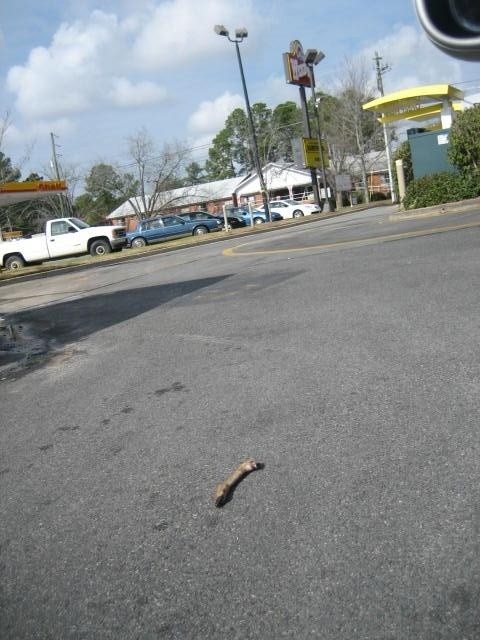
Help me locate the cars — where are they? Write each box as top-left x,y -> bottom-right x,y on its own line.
254,199 -> 322,219
217,205 -> 284,225
177,211 -> 247,232
126,214 -> 223,249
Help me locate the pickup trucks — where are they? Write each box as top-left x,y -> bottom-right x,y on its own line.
0,216 -> 129,272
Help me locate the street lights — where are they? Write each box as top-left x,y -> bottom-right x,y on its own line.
303,47 -> 336,213
212,24 -> 271,223
371,51 -> 400,204
